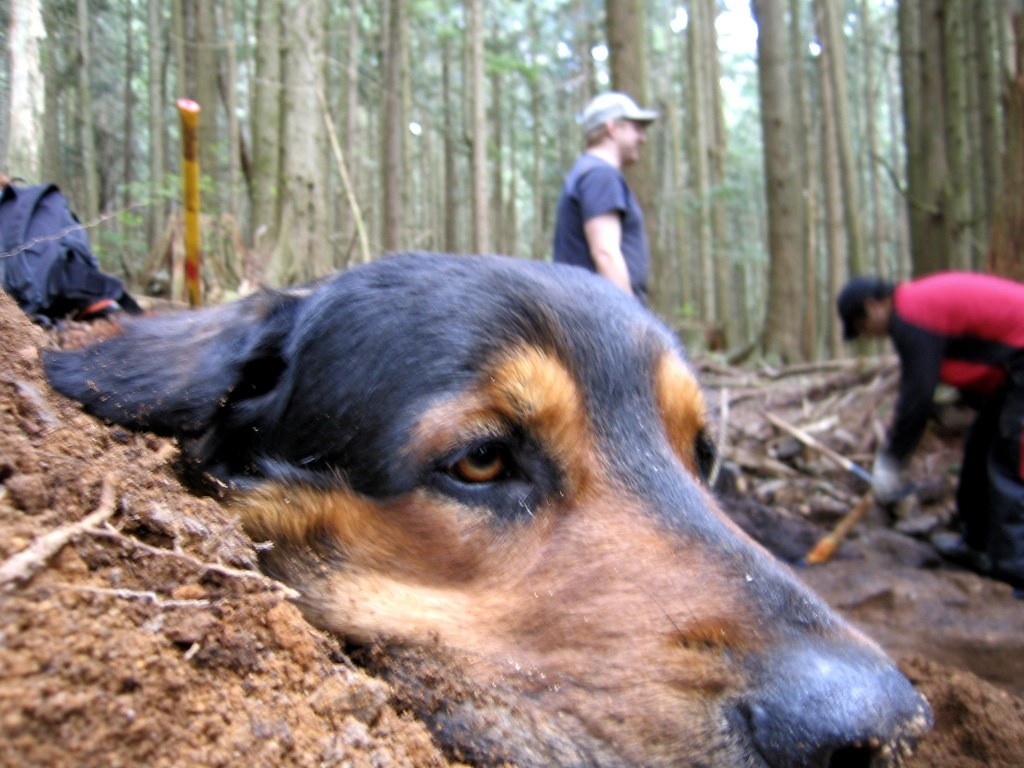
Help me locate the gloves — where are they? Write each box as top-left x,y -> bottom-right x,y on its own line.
872,454 -> 908,507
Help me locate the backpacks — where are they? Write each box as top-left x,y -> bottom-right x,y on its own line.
1,180 -> 100,320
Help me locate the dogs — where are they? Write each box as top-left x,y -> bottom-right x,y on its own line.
32,253 -> 937,768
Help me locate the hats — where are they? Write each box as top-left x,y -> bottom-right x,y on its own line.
836,278 -> 893,339
582,93 -> 655,132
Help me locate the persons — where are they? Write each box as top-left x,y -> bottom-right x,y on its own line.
837,271 -> 1024,600
552,91 -> 658,308
